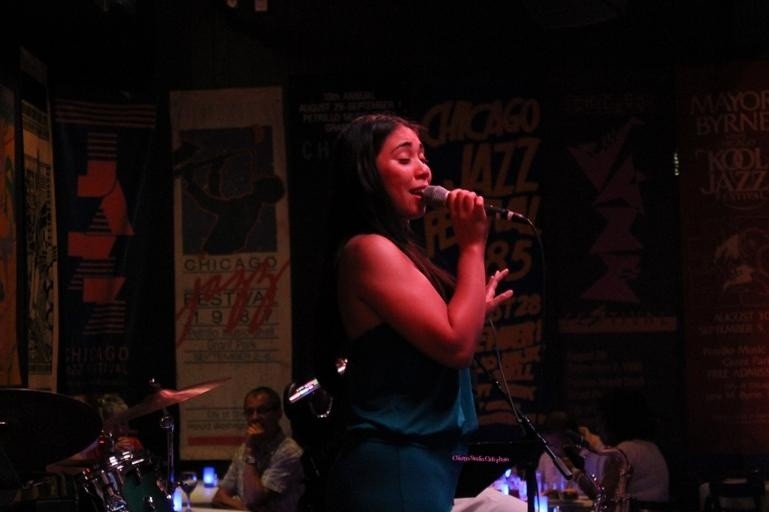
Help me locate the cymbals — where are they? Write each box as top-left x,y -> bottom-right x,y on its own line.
0,388 -> 104,472
123,378 -> 231,421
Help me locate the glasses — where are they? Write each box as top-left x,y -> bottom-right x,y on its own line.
243,405 -> 274,417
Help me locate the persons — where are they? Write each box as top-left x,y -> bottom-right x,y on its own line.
211,385 -> 306,512
97,391 -> 145,455
537,415 -> 602,496
590,391 -> 671,512
296,113 -> 515,512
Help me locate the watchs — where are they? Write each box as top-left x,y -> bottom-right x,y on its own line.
244,455 -> 258,464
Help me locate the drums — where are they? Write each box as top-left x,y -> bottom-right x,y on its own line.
73,451 -> 155,512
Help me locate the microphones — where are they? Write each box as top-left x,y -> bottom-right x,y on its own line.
422,185 -> 525,223
